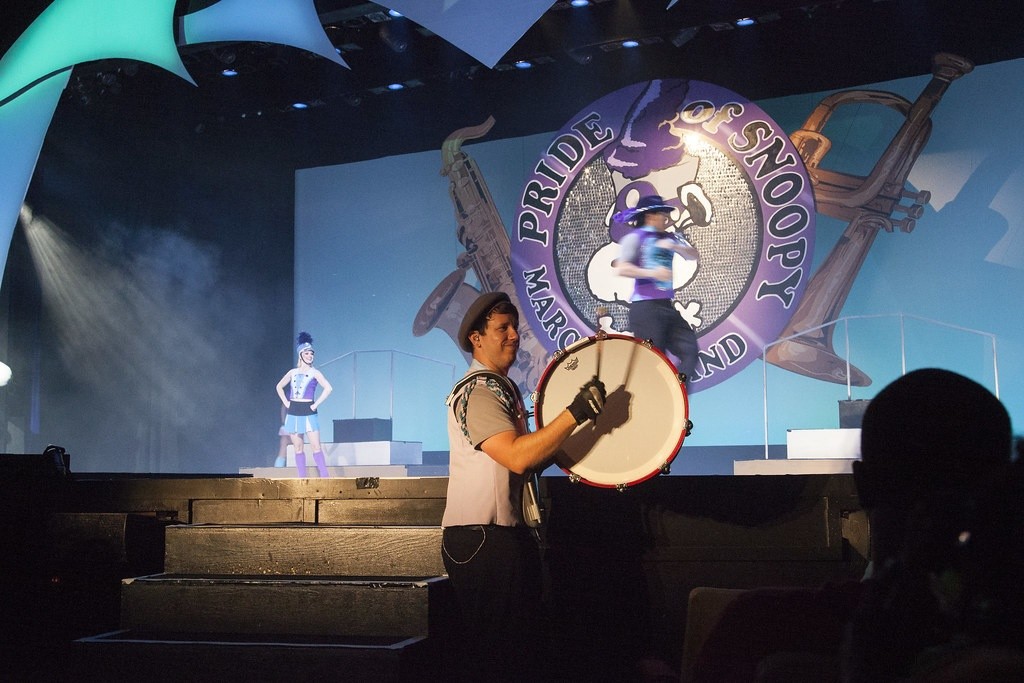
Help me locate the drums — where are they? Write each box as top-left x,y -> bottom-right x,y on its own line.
530,333 -> 694,491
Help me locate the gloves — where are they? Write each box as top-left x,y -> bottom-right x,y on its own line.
565,375 -> 607,427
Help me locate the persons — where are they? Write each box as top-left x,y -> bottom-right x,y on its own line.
619,196 -> 699,383
441,292 -> 605,683
688,368 -> 1024,683
276,332 -> 333,477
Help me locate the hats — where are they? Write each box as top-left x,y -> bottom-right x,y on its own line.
296,330 -> 316,356
621,195 -> 677,222
456,290 -> 512,353
